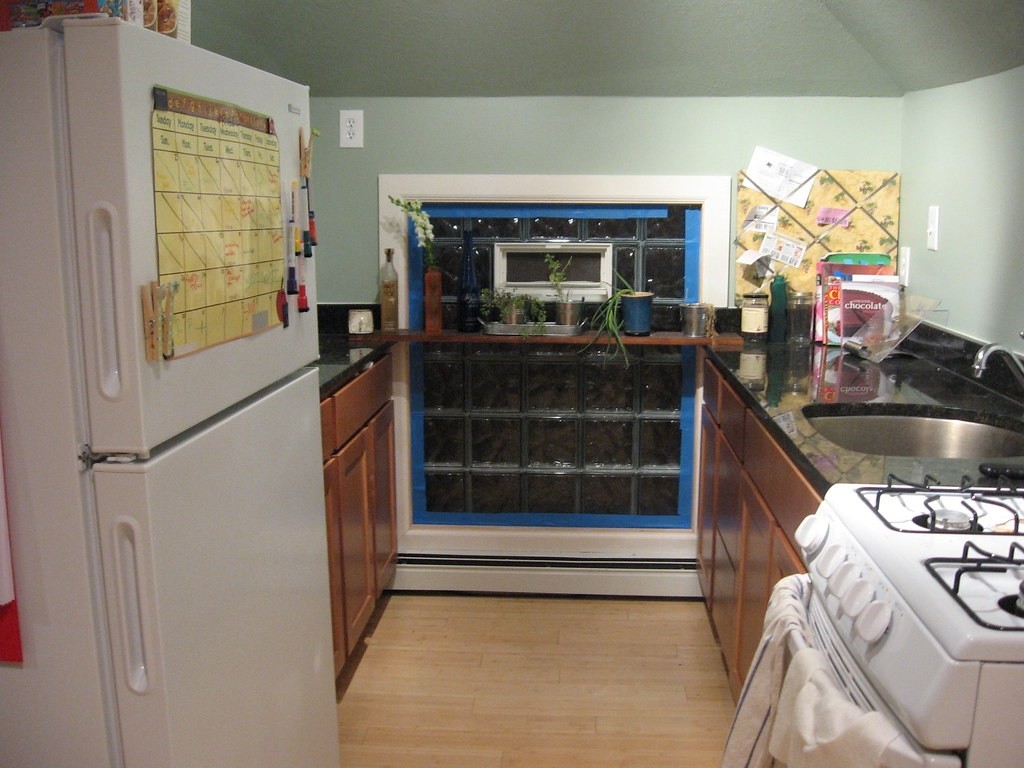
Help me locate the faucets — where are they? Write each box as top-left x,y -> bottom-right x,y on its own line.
971,342 -> 1024,395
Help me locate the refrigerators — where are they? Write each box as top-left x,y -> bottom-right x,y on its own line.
0,13 -> 342,768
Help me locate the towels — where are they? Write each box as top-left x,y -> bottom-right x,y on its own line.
764,648 -> 901,768
720,572 -> 813,768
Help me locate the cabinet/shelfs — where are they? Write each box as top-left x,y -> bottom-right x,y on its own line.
698,358 -> 822,707
411,341 -> 692,529
319,354 -> 398,678
407,201 -> 701,334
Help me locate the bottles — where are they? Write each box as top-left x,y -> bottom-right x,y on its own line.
441,296 -> 458,329
458,219 -> 480,333
770,276 -> 787,342
790,292 -> 814,345
741,293 -> 768,344
736,345 -> 766,392
380,249 -> 398,332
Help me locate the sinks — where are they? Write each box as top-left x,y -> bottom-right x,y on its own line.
795,403 -> 1024,457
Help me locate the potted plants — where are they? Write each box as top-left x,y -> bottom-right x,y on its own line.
578,268 -> 654,368
544,253 -> 582,324
481,288 -> 547,339
388,195 -> 443,333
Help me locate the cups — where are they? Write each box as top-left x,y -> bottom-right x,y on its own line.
348,309 -> 374,333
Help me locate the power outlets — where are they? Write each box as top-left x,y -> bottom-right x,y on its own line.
338,109 -> 363,148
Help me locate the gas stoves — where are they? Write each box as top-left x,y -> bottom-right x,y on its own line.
834,463 -> 1024,748
